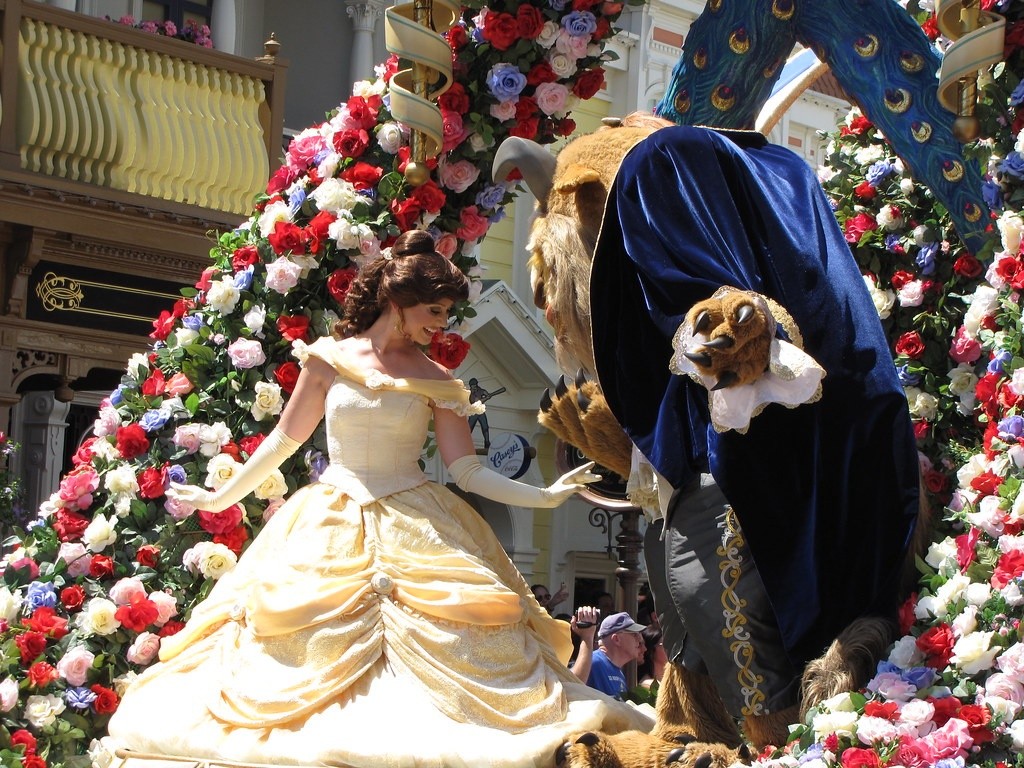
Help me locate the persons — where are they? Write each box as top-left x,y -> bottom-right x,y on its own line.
529,581 -> 671,707
91,230 -> 667,767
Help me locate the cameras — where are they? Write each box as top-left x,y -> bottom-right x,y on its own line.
574,609 -> 601,628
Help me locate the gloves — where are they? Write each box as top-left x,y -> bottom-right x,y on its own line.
445,454 -> 603,509
164,424 -> 303,514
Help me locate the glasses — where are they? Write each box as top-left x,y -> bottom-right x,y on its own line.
535,593 -> 552,603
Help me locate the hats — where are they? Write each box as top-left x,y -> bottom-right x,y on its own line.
595,611 -> 647,642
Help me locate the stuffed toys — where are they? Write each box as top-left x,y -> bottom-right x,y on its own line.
493,110 -> 936,768
